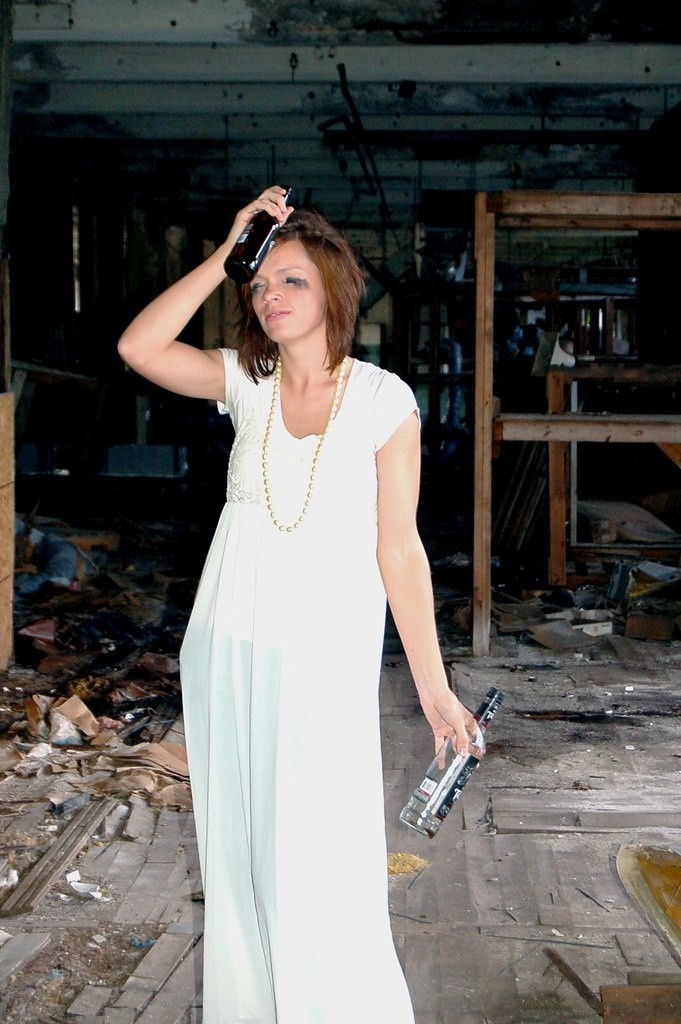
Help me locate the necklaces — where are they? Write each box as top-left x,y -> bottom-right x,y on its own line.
261,355 -> 347,532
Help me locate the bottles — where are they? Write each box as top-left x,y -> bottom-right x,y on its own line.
399,687 -> 506,838
224,185 -> 293,284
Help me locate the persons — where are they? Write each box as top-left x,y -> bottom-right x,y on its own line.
116,186 -> 486,1024
